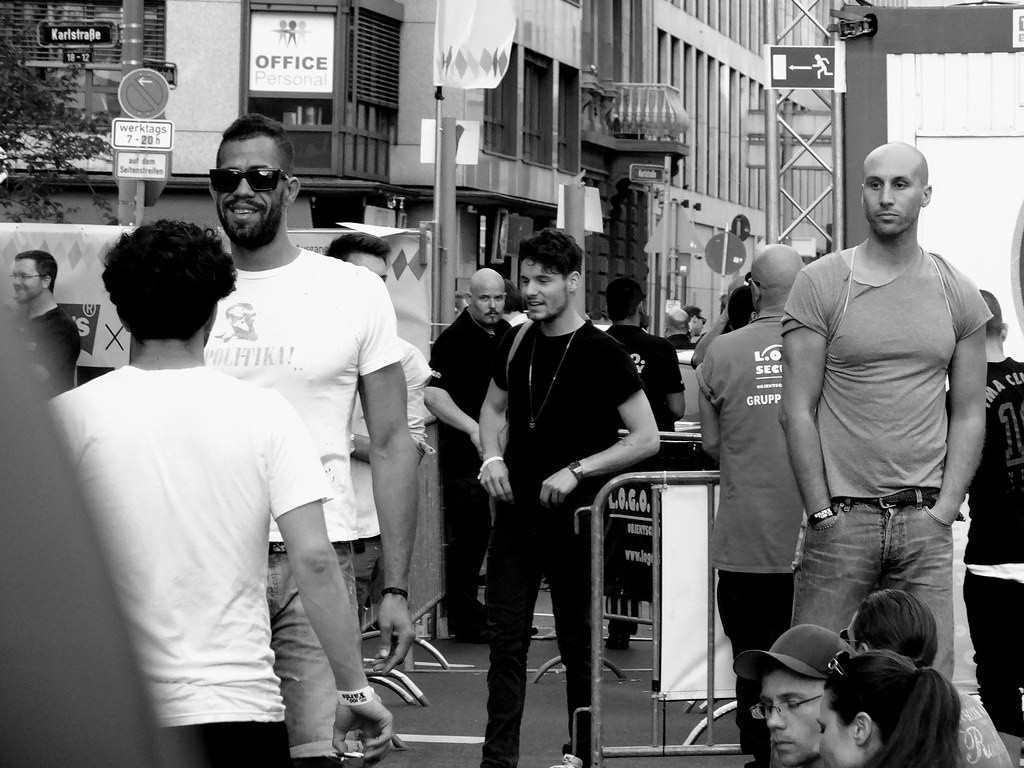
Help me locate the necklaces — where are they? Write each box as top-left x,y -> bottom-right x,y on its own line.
528,325 -> 576,429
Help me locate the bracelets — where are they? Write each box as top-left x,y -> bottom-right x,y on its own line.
808,506 -> 837,526
382,586 -> 408,598
338,684 -> 376,708
478,456 -> 505,480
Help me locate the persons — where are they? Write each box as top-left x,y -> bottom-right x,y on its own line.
479,227 -> 660,768
601,277 -> 687,653
50,220 -> 394,768
665,242 -> 820,768
199,115 -> 415,768
946,288 -> 1024,735
424,268 -> 539,643
322,230 -> 434,632
839,589 -> 1014,768
816,648 -> 960,768
778,141 -> 995,684
733,623 -> 859,768
7,249 -> 82,405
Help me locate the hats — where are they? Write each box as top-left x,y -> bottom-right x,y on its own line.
733,624 -> 859,678
605,279 -> 646,310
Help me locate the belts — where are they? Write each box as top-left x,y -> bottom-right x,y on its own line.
268,541 -> 350,555
830,489 -> 964,522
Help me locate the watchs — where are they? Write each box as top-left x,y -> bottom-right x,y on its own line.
569,461 -> 584,482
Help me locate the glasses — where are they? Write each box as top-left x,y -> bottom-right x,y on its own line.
691,314 -> 706,325
7,273 -> 46,280
209,167 -> 289,193
744,272 -> 760,287
749,695 -> 821,719
840,628 -> 872,650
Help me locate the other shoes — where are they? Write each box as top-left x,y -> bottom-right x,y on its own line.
550,753 -> 582,768
601,632 -> 630,648
478,573 -> 489,588
457,618 -> 538,644
